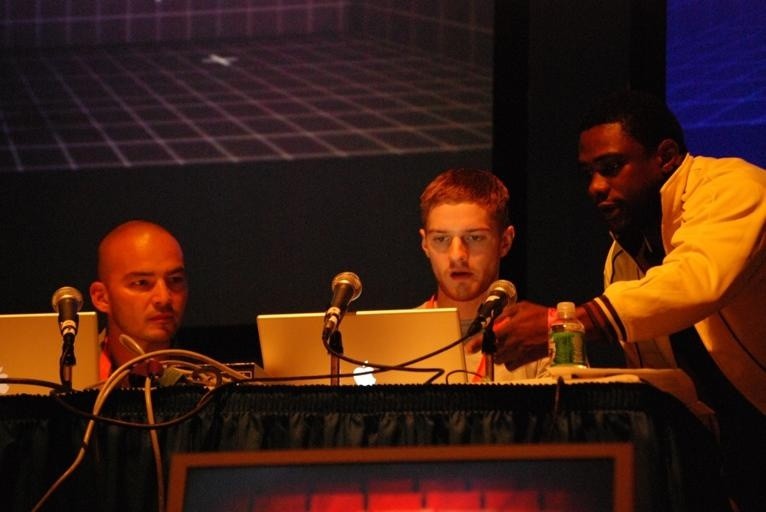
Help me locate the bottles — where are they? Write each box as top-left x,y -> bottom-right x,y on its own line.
549,301 -> 584,365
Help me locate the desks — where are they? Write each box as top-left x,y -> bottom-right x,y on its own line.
0,381 -> 733,511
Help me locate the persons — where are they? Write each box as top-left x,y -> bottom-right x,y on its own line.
467,98 -> 766,512
88,220 -> 188,394
409,164 -> 552,384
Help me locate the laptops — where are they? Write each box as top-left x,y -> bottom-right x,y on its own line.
0,311 -> 100,396
255,308 -> 468,385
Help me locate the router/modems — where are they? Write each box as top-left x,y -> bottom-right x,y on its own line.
167,363 -> 262,386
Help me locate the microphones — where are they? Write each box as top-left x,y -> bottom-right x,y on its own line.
322,271 -> 363,333
52,285 -> 84,364
469,280 -> 517,336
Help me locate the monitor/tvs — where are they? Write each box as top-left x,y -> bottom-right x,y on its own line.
164,443 -> 633,512
